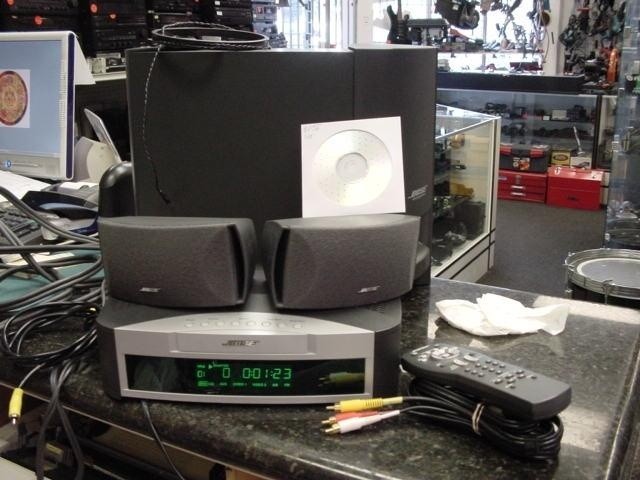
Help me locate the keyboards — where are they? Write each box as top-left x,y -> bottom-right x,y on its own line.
0,205 -> 60,250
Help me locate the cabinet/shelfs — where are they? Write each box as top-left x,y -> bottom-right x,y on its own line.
430,103 -> 502,283
435,86 -> 637,206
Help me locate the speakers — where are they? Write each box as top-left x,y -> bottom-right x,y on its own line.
97,215 -> 259,309
261,213 -> 422,311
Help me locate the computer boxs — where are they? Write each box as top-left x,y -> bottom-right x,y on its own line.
124,46 -> 437,286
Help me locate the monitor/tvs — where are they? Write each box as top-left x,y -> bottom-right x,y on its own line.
1,31 -> 75,181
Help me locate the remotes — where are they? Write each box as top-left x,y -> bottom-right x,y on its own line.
402,342 -> 572,420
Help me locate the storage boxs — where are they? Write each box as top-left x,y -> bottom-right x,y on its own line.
497,170 -> 548,204
546,166 -> 604,210
499,143 -> 550,173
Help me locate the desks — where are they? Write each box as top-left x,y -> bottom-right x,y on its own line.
0,274 -> 640,480
